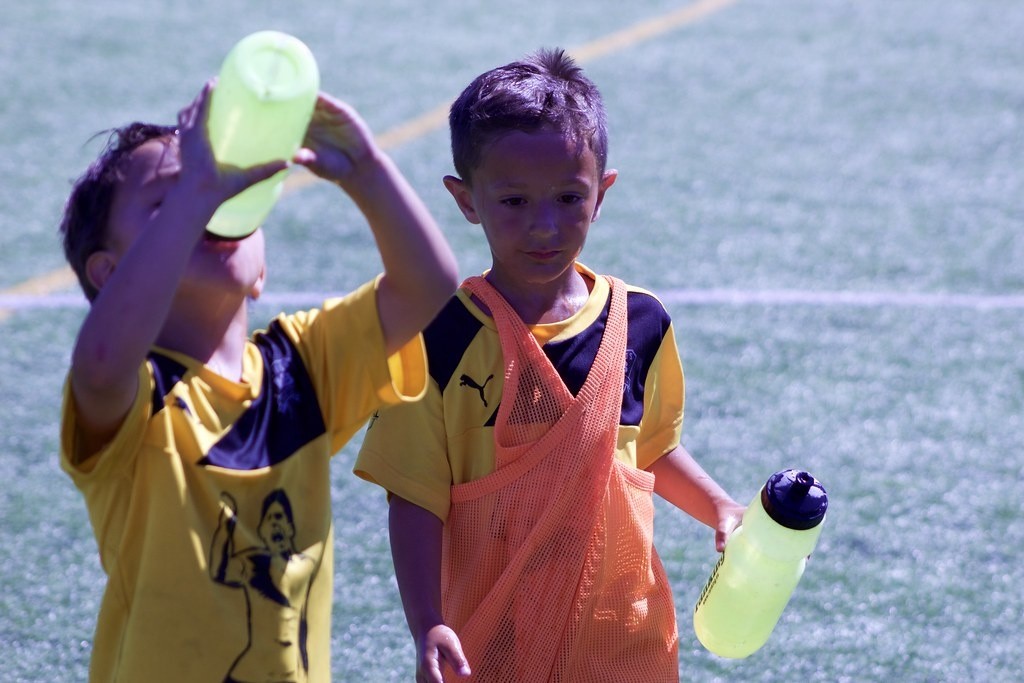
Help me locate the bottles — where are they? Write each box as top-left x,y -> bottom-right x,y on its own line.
206,30 -> 321,242
693,469 -> 830,659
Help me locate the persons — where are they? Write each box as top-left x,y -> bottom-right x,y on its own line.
354,47 -> 750,683
57,81 -> 459,679
